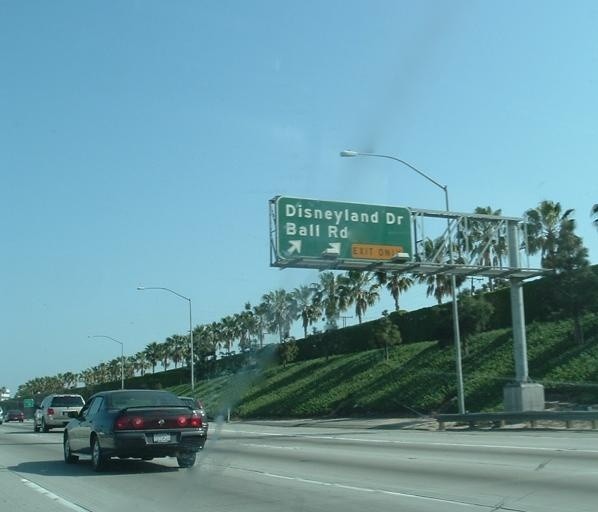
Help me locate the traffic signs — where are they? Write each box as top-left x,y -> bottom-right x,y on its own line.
276,195 -> 413,269
24,399 -> 34,408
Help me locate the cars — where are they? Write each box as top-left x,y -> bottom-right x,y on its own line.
0,406 -> 5,424
4,409 -> 25,423
176,396 -> 209,452
63,387 -> 204,470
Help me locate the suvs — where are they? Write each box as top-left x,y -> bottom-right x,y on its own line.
33,393 -> 86,432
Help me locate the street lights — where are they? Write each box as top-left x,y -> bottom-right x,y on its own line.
340,148 -> 468,420
136,286 -> 195,398
87,335 -> 125,389
